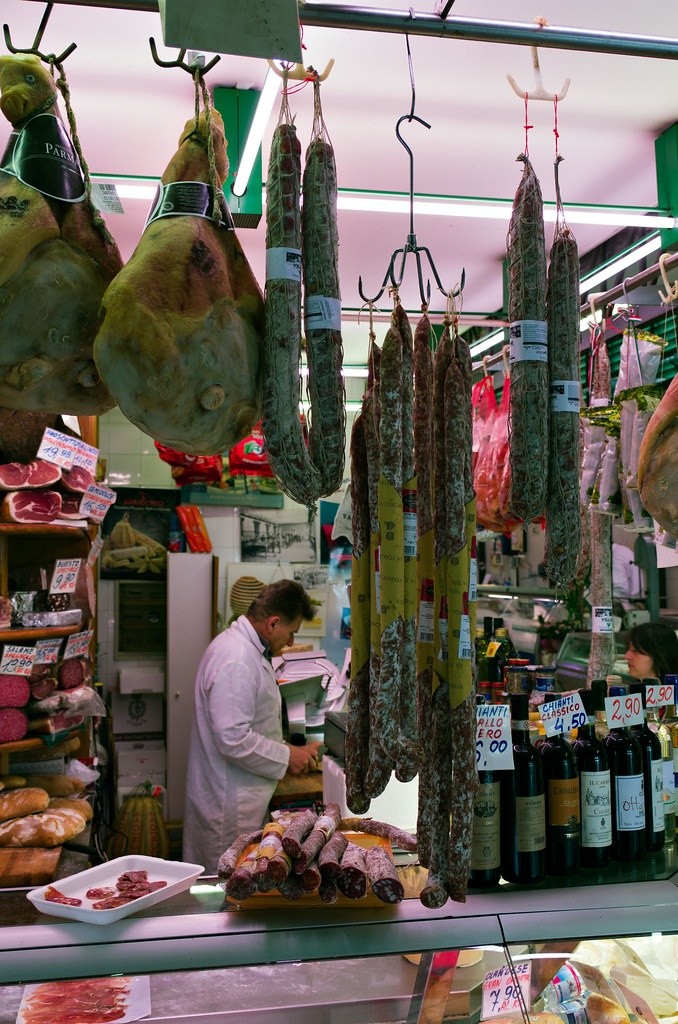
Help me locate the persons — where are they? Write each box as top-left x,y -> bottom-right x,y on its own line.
612,541 -> 643,598
183,579 -> 325,876
624,623 -> 678,678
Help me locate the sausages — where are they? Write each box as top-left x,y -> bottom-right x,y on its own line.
262,123 -> 477,909
216,803 -> 423,904
504,152 -> 614,690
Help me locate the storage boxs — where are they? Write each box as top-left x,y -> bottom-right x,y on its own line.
118,667 -> 165,693
114,741 -> 167,788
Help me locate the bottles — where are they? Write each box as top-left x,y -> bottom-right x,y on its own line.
539,960 -> 603,1024
471,628 -> 678,887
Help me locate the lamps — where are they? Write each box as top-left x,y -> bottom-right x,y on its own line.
232,59 -> 293,197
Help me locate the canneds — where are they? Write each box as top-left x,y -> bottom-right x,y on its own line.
477,658 -> 556,708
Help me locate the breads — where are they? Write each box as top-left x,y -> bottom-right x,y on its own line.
476,993 -> 632,1024
0,772 -> 94,848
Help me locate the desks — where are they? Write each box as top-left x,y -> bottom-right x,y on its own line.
272,772 -> 323,805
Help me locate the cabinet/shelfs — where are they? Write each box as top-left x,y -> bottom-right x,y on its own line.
0,498 -> 97,775
